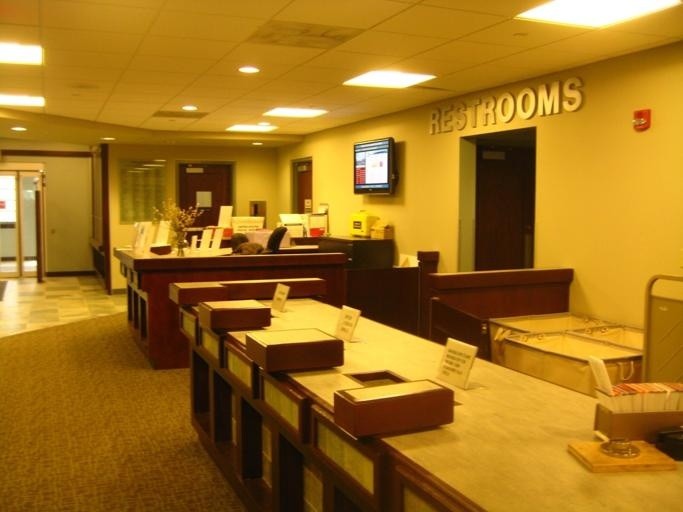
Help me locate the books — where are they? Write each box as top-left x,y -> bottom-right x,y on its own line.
566,438 -> 680,474
593,381 -> 683,415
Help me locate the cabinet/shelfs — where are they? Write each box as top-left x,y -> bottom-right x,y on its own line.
292,234 -> 393,268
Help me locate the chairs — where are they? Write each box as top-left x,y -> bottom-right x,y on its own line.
266,226 -> 288,253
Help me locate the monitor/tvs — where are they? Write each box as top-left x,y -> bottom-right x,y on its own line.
353,134 -> 399,196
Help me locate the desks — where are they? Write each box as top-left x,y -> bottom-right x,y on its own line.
177,296 -> 682,512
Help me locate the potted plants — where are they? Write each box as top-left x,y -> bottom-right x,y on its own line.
151,198 -> 204,257
